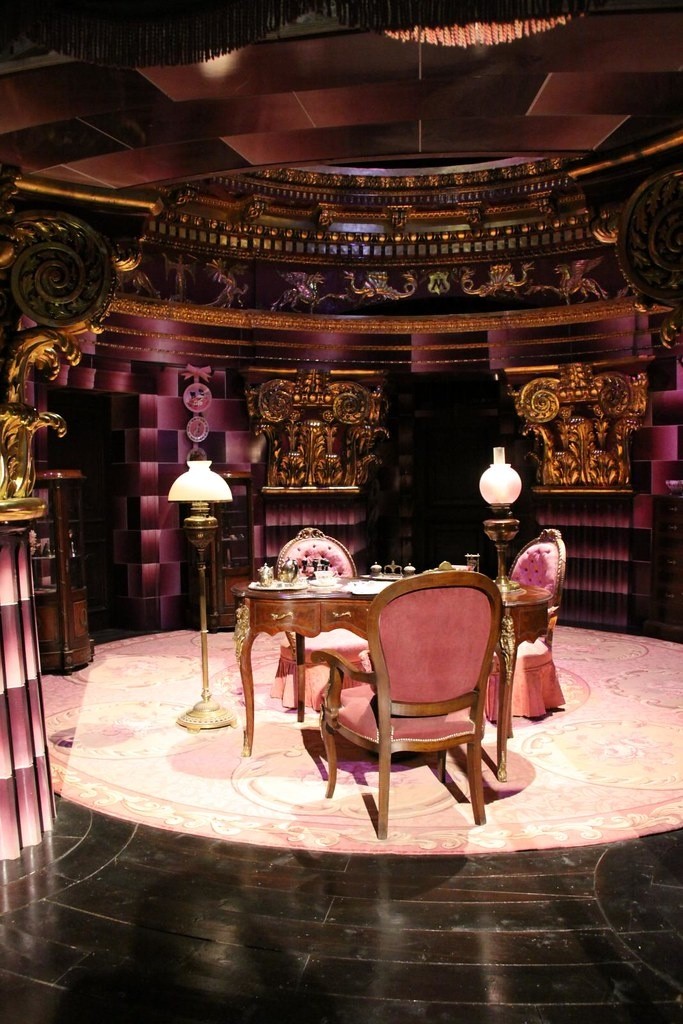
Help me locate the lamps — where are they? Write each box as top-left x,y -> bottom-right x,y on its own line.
167,458 -> 240,731
479,445 -> 525,599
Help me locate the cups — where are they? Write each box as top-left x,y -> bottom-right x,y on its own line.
403,563 -> 416,577
315,571 -> 333,586
370,562 -> 382,576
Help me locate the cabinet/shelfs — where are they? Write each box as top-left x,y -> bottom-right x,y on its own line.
30,467 -> 93,673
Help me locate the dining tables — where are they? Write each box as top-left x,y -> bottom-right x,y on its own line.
228,573 -> 556,785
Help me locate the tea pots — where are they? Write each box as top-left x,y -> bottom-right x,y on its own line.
258,563 -> 274,586
280,557 -> 299,585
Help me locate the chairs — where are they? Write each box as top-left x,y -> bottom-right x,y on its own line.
483,527 -> 568,722
269,526 -> 371,712
308,569 -> 504,842
